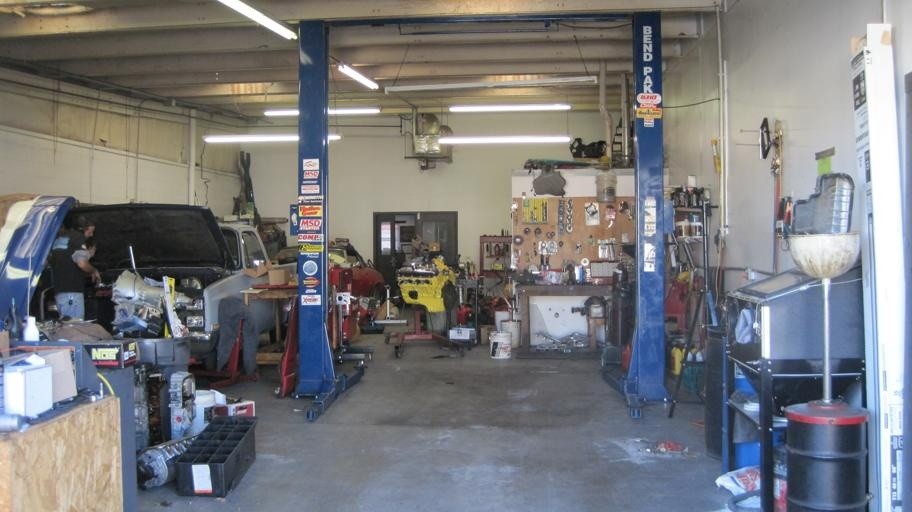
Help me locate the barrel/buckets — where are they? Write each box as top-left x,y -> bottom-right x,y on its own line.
704,325 -> 733,461
722,359 -> 785,472
596,173 -> 617,202
489,331 -> 512,359
502,320 -> 521,349
192,390 -> 216,436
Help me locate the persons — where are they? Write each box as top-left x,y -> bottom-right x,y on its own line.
48,214 -> 102,320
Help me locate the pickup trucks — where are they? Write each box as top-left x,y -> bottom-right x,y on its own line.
6,202 -> 287,381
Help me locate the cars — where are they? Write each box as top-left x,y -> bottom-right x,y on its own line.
266,236 -> 387,327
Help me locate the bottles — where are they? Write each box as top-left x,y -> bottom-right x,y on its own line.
775,195 -> 793,234
668,343 -> 705,376
671,186 -> 705,237
22,316 -> 40,341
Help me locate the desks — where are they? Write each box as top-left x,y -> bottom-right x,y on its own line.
511,280 -> 621,361
